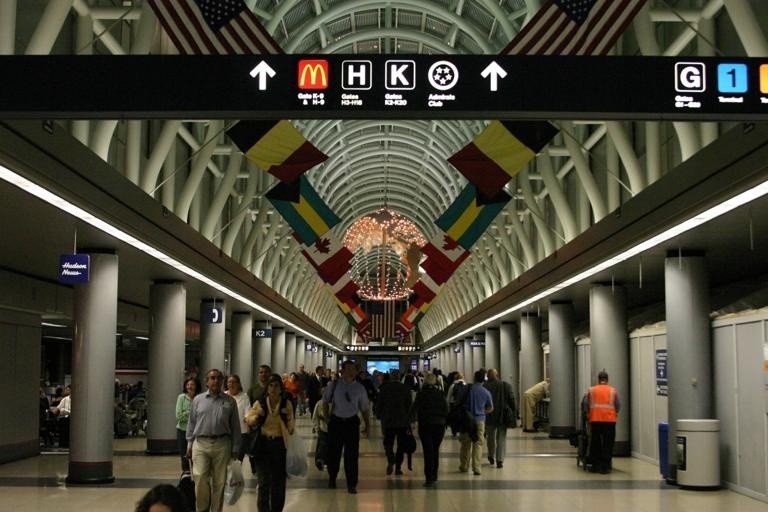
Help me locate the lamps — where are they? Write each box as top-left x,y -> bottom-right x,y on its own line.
342,128 -> 429,302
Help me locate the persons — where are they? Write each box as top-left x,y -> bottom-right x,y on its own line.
176,364 -> 274,480
288,360 -> 518,492
135,481 -> 192,511
113,380 -> 147,436
244,374 -> 295,511
39,379 -> 71,447
580,370 -> 621,473
523,378 -> 551,431
187,369 -> 242,510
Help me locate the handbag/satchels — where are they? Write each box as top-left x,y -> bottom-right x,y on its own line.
503,403 -> 516,428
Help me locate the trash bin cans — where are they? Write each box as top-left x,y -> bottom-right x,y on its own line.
675,418 -> 721,489
658,421 -> 668,479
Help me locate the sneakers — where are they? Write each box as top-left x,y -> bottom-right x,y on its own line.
329,457 -> 503,493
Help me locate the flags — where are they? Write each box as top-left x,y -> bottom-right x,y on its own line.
396,115 -> 558,339
226,119 -> 371,342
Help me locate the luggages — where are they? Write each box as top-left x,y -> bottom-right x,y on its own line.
179,456 -> 211,512
